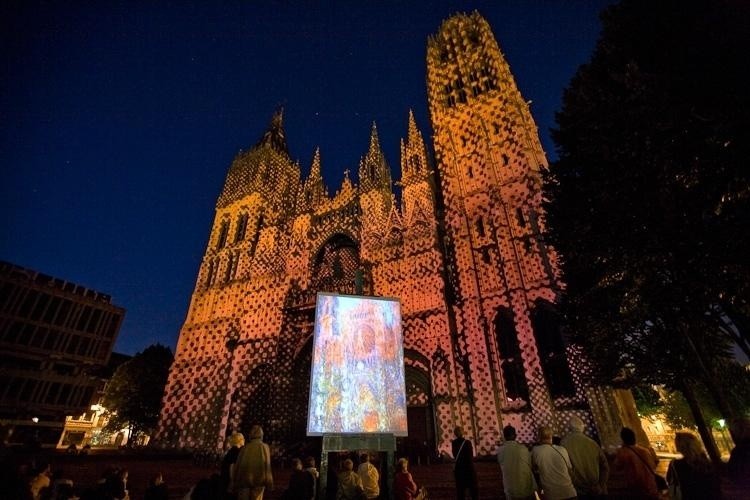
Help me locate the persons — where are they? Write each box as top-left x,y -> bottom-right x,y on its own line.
666,433 -> 714,500
285,454 -> 418,499
531,428 -> 577,500
498,426 -> 534,499
452,427 -> 479,500
726,418 -> 750,485
560,419 -> 610,500
17,417 -> 274,500
613,426 -> 658,500
